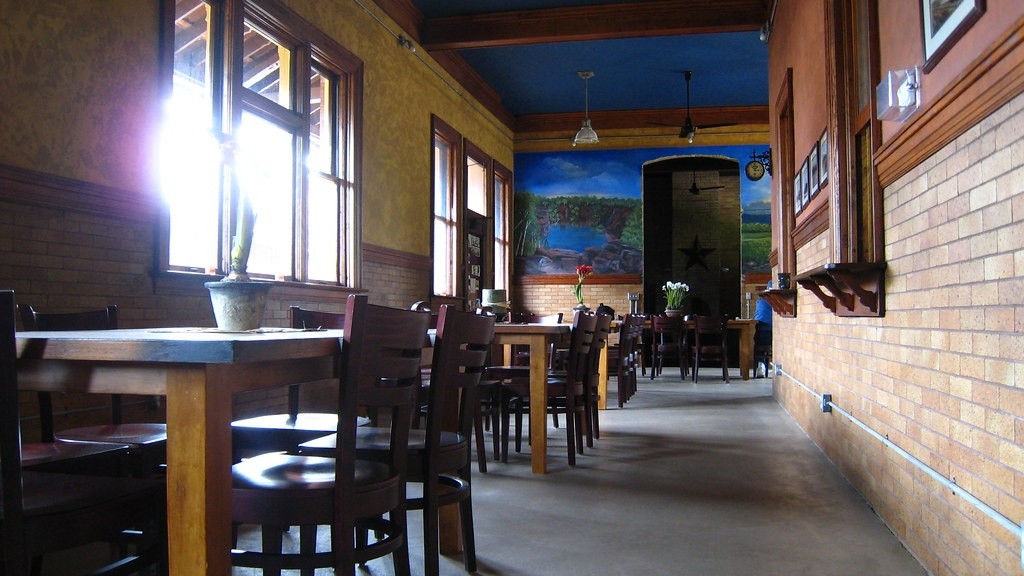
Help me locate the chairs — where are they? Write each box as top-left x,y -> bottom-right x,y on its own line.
0,289 -> 773,576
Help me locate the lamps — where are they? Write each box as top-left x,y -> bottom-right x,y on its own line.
574,70 -> 599,144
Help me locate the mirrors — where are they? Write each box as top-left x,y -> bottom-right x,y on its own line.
641,153 -> 742,322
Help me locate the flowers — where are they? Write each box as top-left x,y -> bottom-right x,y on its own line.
661,282 -> 690,310
573,264 -> 593,303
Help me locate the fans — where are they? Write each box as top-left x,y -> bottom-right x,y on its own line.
652,70 -> 739,141
671,164 -> 725,195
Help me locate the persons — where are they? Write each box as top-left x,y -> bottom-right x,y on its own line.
753,277 -> 771,367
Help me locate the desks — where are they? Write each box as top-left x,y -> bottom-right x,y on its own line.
641,318 -> 757,381
0,324 -> 354,576
477,321 -> 574,474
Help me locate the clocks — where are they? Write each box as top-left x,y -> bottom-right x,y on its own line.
745,161 -> 765,181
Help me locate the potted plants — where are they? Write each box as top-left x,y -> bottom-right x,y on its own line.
205,206 -> 275,331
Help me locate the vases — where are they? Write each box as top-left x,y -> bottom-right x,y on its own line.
664,310 -> 680,318
572,305 -> 589,317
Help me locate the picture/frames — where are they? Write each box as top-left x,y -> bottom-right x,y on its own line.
794,126 -> 829,218
919,0 -> 986,74
